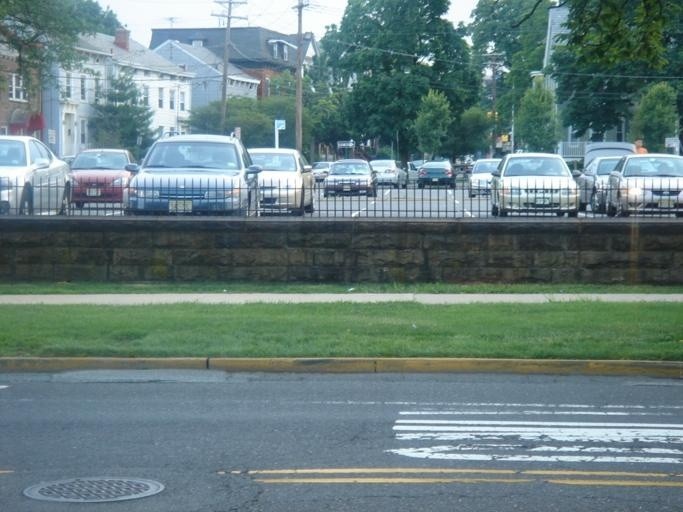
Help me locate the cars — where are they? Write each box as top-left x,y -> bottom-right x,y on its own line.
368,159 -> 408,188
0,135 -> 72,215
489,152 -> 581,218
321,159 -> 378,198
416,162 -> 456,189
466,158 -> 503,199
569,142 -> 638,215
605,153 -> 682,218
70,147 -> 140,209
241,148 -> 316,216
406,161 -> 419,182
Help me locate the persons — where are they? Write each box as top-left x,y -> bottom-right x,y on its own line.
633,137 -> 648,154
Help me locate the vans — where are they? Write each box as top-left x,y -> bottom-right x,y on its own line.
312,161 -> 335,180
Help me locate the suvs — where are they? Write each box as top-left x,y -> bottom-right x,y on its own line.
125,132 -> 261,218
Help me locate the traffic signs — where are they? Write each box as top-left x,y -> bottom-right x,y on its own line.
337,141 -> 354,148
